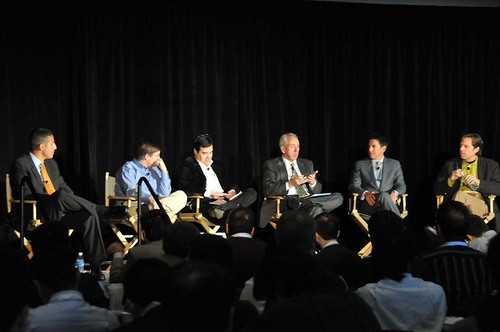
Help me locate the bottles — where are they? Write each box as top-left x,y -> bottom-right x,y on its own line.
75,251 -> 84,272
461,173 -> 479,191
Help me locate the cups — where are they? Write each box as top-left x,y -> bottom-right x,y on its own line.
108,282 -> 124,308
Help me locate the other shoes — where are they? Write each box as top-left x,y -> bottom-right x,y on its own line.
423,225 -> 439,240
208,205 -> 224,220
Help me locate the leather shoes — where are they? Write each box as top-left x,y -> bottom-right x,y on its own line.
91,267 -> 105,280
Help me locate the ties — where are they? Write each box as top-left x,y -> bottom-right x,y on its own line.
40,163 -> 56,195
375,161 -> 381,189
291,163 -> 309,197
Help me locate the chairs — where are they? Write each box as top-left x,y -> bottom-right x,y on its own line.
264,195 -> 283,229
105,172 -> 143,271
436,194 -> 496,224
6,173 -> 75,260
177,196 -> 221,235
348,192 -> 409,257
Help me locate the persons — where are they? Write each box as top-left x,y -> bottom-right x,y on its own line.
0,200 -> 500,332
434,133 -> 500,220
348,134 -> 406,218
114,142 -> 187,224
11,127 -> 107,282
177,132 -> 258,233
264,132 -> 343,219
